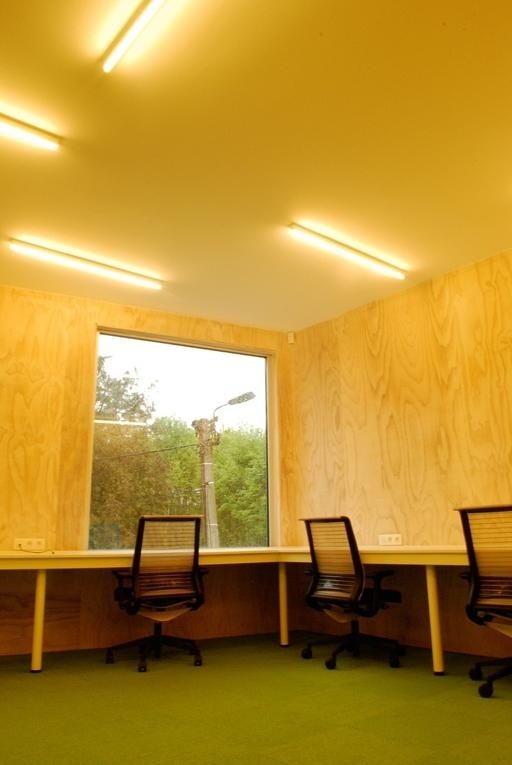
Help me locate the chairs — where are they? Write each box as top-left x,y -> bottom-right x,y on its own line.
454,505 -> 512,699
300,515 -> 405,669
104,515 -> 209,672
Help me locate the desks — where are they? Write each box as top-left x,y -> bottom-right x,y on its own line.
1,546 -> 512,676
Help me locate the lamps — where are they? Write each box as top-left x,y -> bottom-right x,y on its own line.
287,224 -> 405,279
102,0 -> 166,73
10,237 -> 161,290
0,114 -> 61,151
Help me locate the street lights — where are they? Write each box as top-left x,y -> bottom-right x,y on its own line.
190,390 -> 258,549
